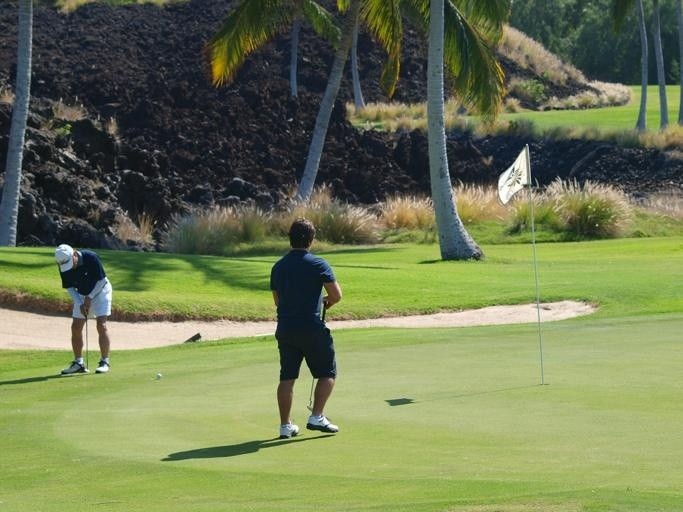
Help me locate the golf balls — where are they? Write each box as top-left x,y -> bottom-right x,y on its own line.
157,374 -> 161,378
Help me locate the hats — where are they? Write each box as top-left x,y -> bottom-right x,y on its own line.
54,243 -> 74,273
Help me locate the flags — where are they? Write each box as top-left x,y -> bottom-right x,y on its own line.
495,144 -> 530,207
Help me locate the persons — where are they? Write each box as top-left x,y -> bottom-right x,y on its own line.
53,242 -> 113,378
268,213 -> 344,440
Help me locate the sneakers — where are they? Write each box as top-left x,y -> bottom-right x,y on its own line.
95,360 -> 110,373
306,415 -> 339,433
61,361 -> 85,373
280,424 -> 299,438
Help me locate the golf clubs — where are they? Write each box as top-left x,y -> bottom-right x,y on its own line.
83,315 -> 90,372
306,301 -> 327,410
